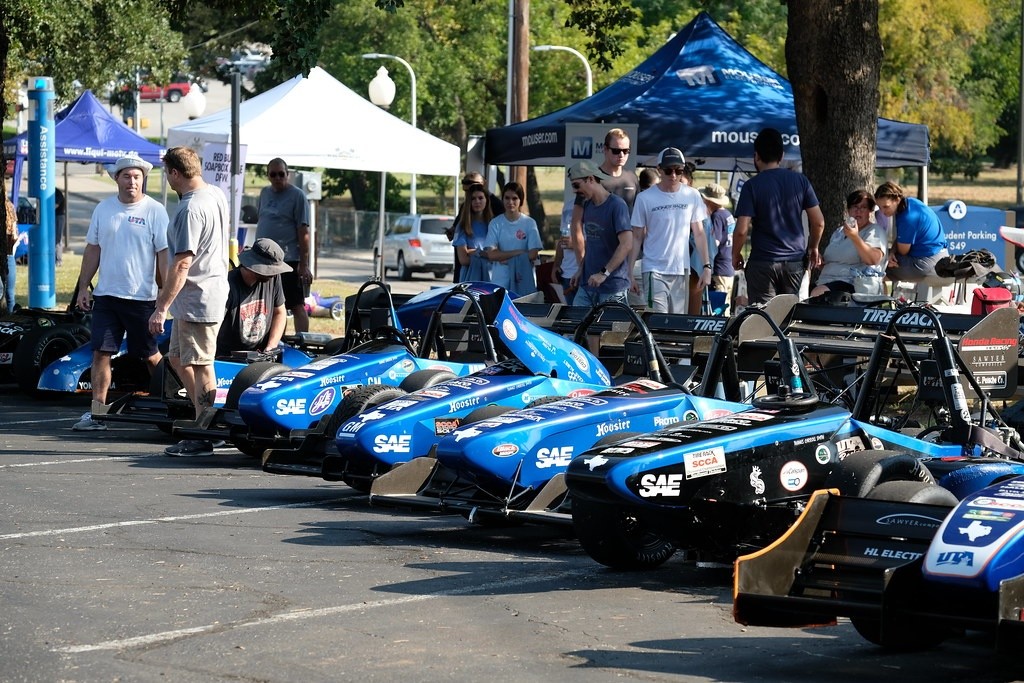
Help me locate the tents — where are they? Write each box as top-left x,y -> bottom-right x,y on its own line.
164,66 -> 461,285
483,9 -> 929,202
3,89 -> 168,250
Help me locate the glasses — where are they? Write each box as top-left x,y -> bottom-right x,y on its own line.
462,178 -> 480,185
851,205 -> 870,212
572,178 -> 587,189
269,171 -> 285,178
663,168 -> 684,175
609,147 -> 630,155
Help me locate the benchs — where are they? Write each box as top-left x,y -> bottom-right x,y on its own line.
903,275 -> 982,305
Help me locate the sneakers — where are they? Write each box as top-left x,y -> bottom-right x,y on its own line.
72,412 -> 107,431
164,439 -> 225,456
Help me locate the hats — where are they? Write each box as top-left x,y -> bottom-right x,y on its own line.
658,147 -> 685,169
239,238 -> 294,276
567,160 -> 613,181
108,155 -> 153,180
697,183 -> 729,205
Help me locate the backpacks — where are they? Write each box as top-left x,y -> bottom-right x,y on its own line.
935,249 -> 996,279
971,287 -> 1012,315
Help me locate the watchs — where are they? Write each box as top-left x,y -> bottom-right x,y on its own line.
601,266 -> 610,277
703,263 -> 712,270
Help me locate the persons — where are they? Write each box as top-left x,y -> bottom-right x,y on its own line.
0,179 -> 18,318
114,63 -> 137,128
55,187 -> 66,267
810,181 -> 955,299
440,128 -> 737,358
238,245 -> 345,320
15,196 -> 36,265
731,126 -> 824,309
238,227 -> 248,260
216,238 -> 293,362
71,154 -> 170,430
149,146 -> 230,457
255,157 -> 311,334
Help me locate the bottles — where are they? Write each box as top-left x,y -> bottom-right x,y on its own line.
846,217 -> 856,228
563,224 -> 571,236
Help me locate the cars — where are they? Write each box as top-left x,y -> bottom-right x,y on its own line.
121,76 -> 190,102
374,214 -> 454,281
71,69 -> 145,98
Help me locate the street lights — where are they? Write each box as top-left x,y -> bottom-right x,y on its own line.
532,45 -> 593,96
369,66 -> 396,276
361,54 -> 417,214
